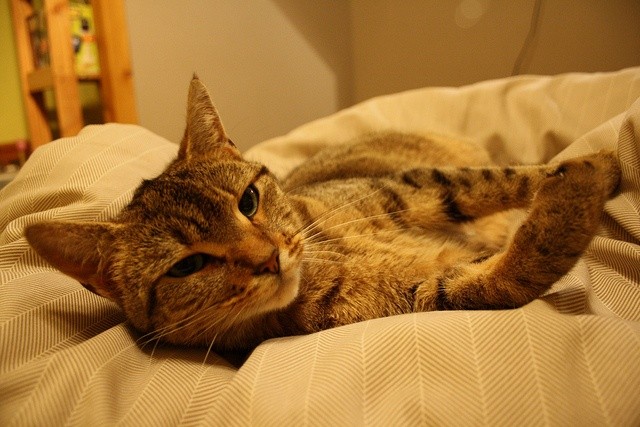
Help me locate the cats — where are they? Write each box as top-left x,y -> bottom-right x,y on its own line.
21,70 -> 621,374
